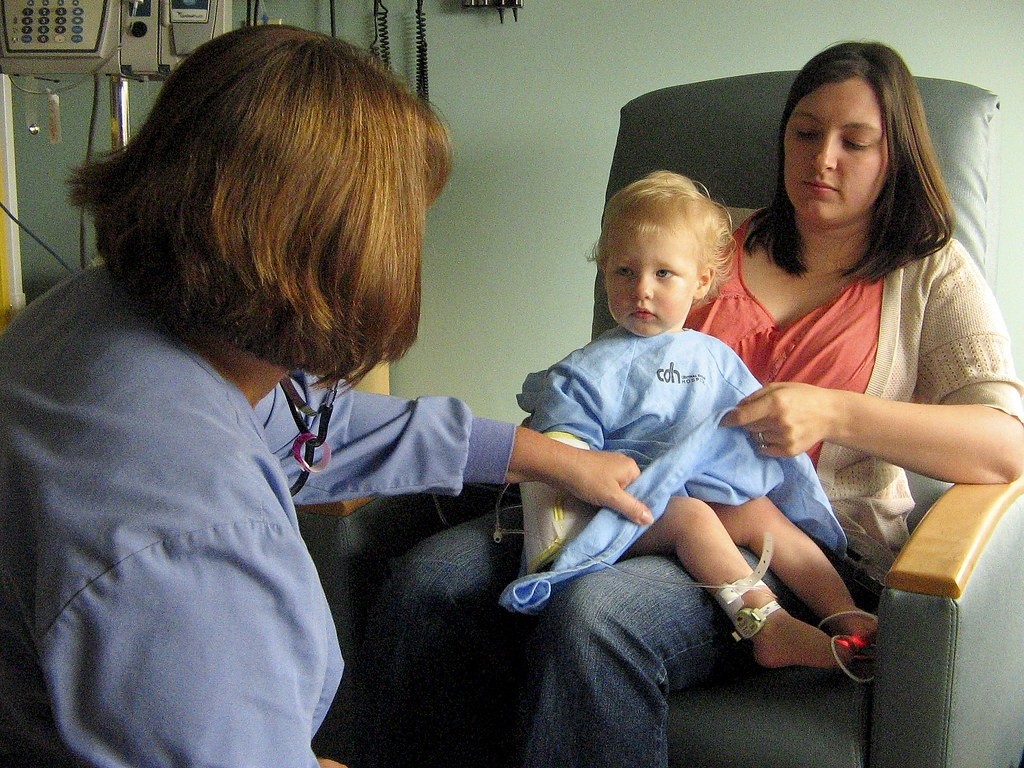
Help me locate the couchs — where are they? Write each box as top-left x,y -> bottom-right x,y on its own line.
295,70 -> 1024,768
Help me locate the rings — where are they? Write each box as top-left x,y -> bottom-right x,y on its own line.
757,432 -> 769,449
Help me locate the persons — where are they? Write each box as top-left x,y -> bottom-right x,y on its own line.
0,26 -> 654,768
499,171 -> 878,668
349,42 -> 1024,768
